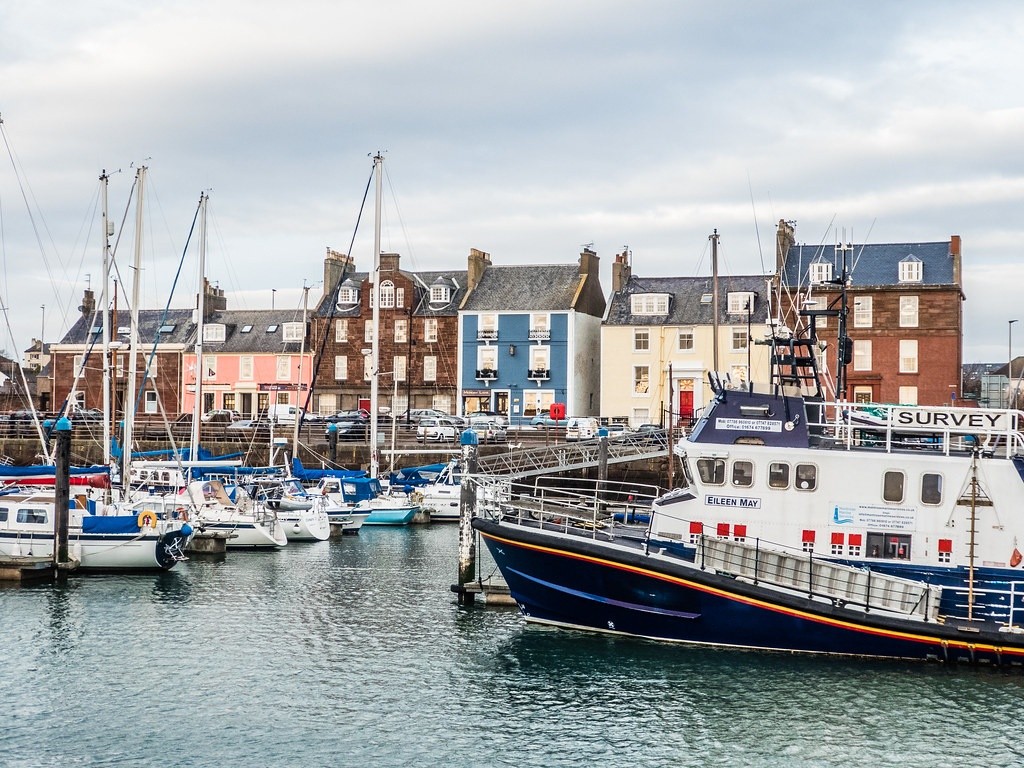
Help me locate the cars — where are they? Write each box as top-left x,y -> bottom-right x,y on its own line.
325,422 -> 370,443
398,409 -> 464,430
200,408 -> 241,426
326,409 -> 369,423
529,412 -> 569,429
607,424 -> 633,442
637,424 -> 662,444
416,418 -> 455,443
225,419 -> 270,429
462,411 -> 509,429
471,421 -> 508,444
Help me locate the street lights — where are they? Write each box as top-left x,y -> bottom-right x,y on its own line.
1007,319 -> 1019,408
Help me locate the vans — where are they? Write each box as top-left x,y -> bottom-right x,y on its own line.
566,418 -> 598,442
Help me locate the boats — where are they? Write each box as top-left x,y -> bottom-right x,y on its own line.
448,200 -> 1024,680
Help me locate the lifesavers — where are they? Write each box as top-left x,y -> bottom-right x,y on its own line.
176,507 -> 188,520
138,510 -> 157,528
413,491 -> 423,502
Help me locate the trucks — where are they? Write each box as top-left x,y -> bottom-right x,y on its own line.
267,403 -> 320,424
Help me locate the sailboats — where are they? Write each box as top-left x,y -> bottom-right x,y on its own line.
0,111 -> 511,574
